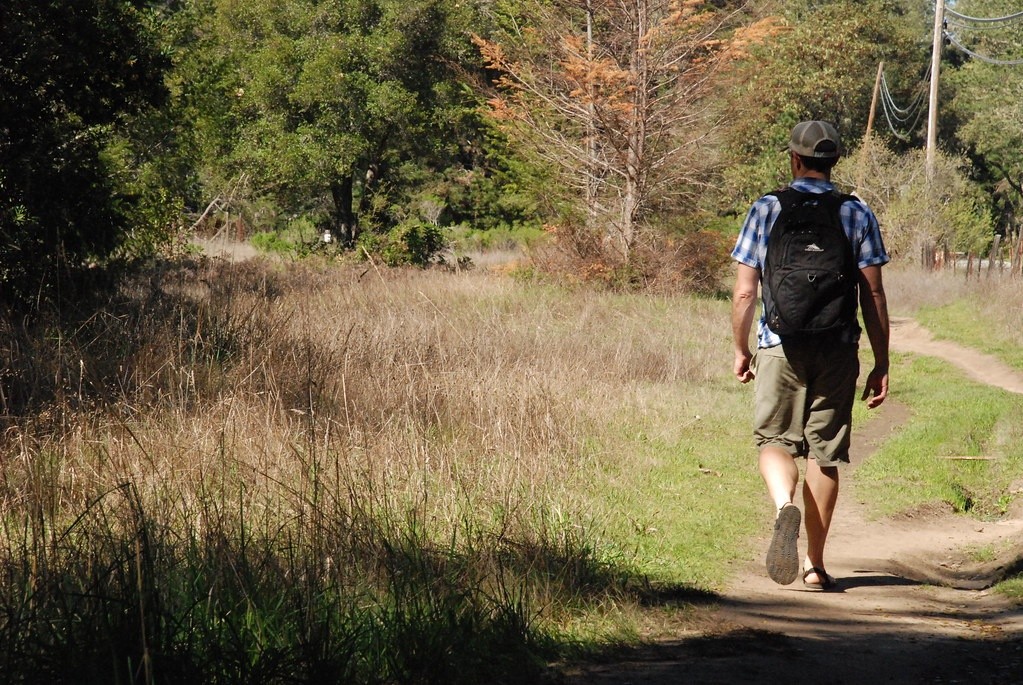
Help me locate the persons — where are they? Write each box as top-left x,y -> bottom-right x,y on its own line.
730,121 -> 890,589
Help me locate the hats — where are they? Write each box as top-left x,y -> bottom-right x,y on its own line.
779,121 -> 841,158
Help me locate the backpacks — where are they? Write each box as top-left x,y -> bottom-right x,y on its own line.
759,187 -> 857,338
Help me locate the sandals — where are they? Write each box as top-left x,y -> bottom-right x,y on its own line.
766,503 -> 802,585
804,568 -> 834,590
